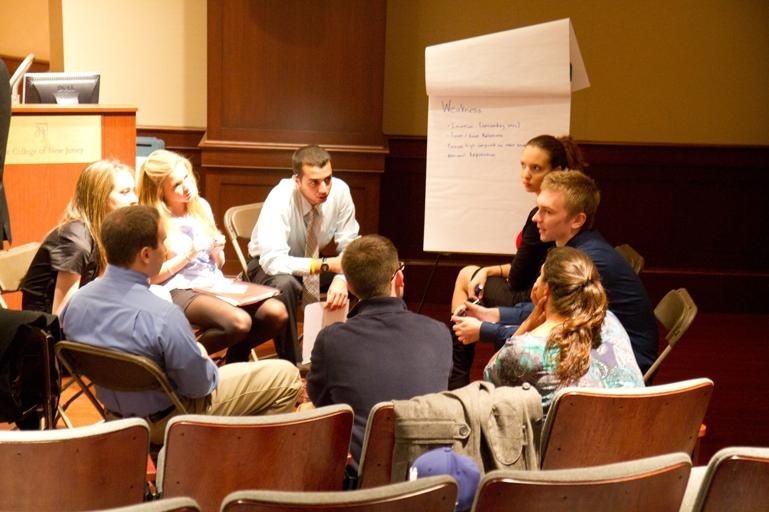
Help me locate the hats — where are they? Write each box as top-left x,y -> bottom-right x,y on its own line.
409,449 -> 481,512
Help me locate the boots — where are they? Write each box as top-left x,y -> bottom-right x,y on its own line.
446,320 -> 476,391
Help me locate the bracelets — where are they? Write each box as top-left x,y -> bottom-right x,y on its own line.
166,259 -> 175,277
499,265 -> 503,278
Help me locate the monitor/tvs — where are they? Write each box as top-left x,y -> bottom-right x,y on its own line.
22,73 -> 100,104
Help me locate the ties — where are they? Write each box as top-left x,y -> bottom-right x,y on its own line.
302,207 -> 320,318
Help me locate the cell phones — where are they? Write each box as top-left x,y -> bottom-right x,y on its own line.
472,298 -> 479,305
449,306 -> 469,317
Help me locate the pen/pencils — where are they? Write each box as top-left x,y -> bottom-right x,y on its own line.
474,284 -> 483,294
450,299 -> 480,322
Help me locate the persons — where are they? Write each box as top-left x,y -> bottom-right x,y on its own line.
0,58 -> 13,313
21,159 -> 139,329
451,170 -> 659,387
247,144 -> 361,365
305,234 -> 452,489
139,149 -> 288,365
485,247 -> 645,417
58,207 -> 302,444
447,135 -> 587,394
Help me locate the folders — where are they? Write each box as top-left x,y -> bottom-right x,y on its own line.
191,276 -> 281,309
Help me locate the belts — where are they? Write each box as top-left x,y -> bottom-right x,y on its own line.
106,401 -> 177,424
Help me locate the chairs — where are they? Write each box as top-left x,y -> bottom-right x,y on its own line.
356,402 -> 395,490
643,288 -> 697,383
680,446 -> 769,512
95,496 -> 202,512
54,340 -> 189,452
0,242 -> 108,431
615,244 -> 645,276
537,378 -> 714,470
1,417 -> 150,512
220,475 -> 458,512
23,325 -> 54,430
224,201 -> 303,362
191,324 -> 209,338
156,404 -> 354,512
471,452 -> 692,512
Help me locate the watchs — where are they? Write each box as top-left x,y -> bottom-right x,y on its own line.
320,256 -> 329,275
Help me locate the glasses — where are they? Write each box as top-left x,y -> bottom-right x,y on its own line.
390,261 -> 404,280
